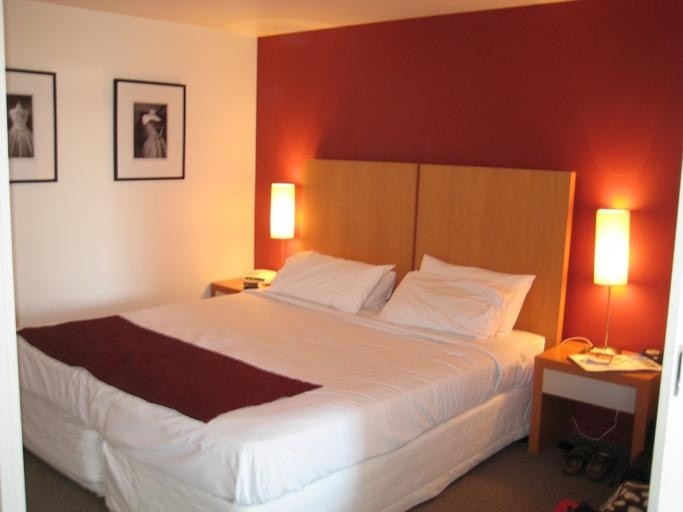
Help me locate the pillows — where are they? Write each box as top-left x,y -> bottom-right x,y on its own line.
420,254 -> 536,339
270,251 -> 398,316
362,271 -> 396,311
381,270 -> 514,342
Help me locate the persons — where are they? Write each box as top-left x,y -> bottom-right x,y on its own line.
8,103 -> 33,158
141,108 -> 166,158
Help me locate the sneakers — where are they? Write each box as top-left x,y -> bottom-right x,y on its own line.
588,445 -> 611,479
565,439 -> 589,477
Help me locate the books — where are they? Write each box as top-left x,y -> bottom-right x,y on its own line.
585,347 -> 614,364
243,279 -> 264,289
567,353 -> 662,373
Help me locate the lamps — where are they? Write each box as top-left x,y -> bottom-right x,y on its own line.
592,207 -> 633,348
267,178 -> 296,265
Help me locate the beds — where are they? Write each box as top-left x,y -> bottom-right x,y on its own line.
15,161 -> 577,511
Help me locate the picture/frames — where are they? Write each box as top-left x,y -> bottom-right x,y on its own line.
110,76 -> 186,183
5,66 -> 57,184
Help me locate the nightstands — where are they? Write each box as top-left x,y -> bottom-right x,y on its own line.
527,339 -> 662,465
209,276 -> 265,296
